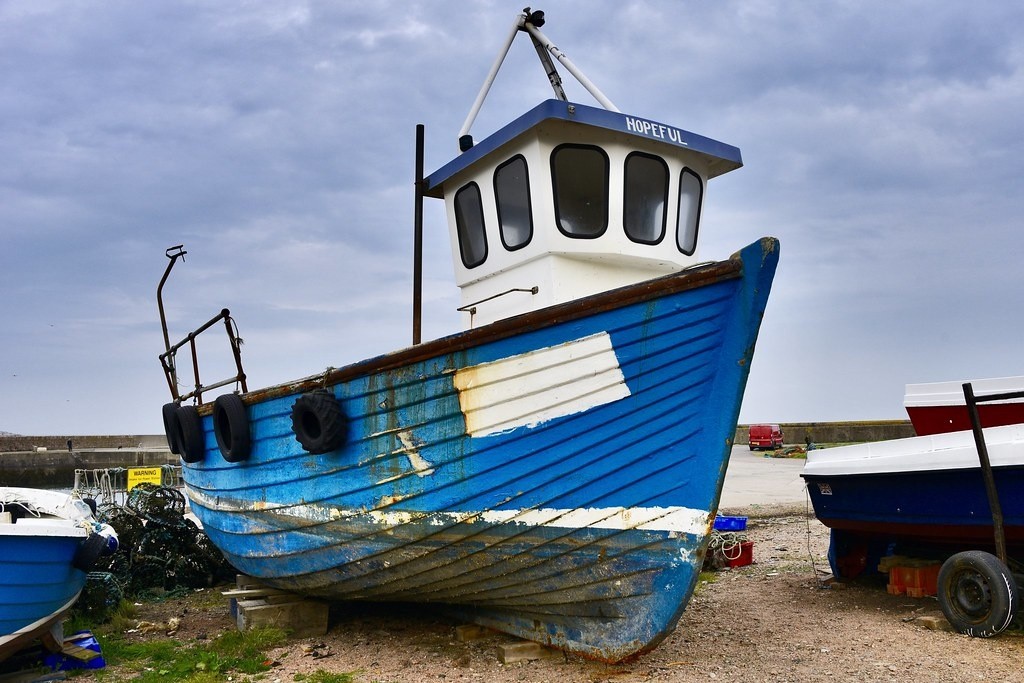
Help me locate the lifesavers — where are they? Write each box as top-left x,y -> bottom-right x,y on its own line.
213,393 -> 251,463
289,391 -> 346,456
83,498 -> 97,515
74,532 -> 108,574
160,403 -> 206,463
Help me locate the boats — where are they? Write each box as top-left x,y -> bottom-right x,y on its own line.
798,416 -> 1024,589
0,485 -> 121,667
903,375 -> 1024,439
154,4 -> 785,667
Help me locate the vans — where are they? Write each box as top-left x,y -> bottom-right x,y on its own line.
748,425 -> 784,450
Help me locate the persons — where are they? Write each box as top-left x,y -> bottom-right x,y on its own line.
804,434 -> 811,447
67,438 -> 73,452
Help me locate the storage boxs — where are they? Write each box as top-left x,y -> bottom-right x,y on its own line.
712,515 -> 748,531
229,587 -> 331,642
887,565 -> 941,596
719,541 -> 756,567
